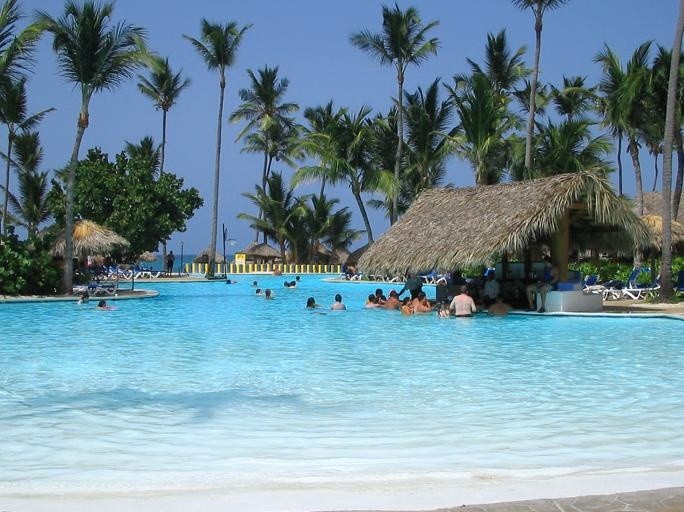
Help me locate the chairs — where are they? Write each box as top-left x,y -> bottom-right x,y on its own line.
84,266 -> 162,296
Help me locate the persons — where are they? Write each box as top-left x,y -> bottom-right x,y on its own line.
166,250 -> 174,276
524,254 -> 559,313
363,269 -> 514,316
330,294 -> 346,310
78,290 -> 89,304
272,265 -> 281,275
283,280 -> 287,287
344,264 -> 356,279
304,296 -> 324,309
287,283 -> 290,287
255,288 -> 261,295
287,281 -> 296,288
296,275 -> 301,282
226,279 -> 232,283
263,288 -> 272,299
95,299 -> 111,311
250,281 -> 258,288
